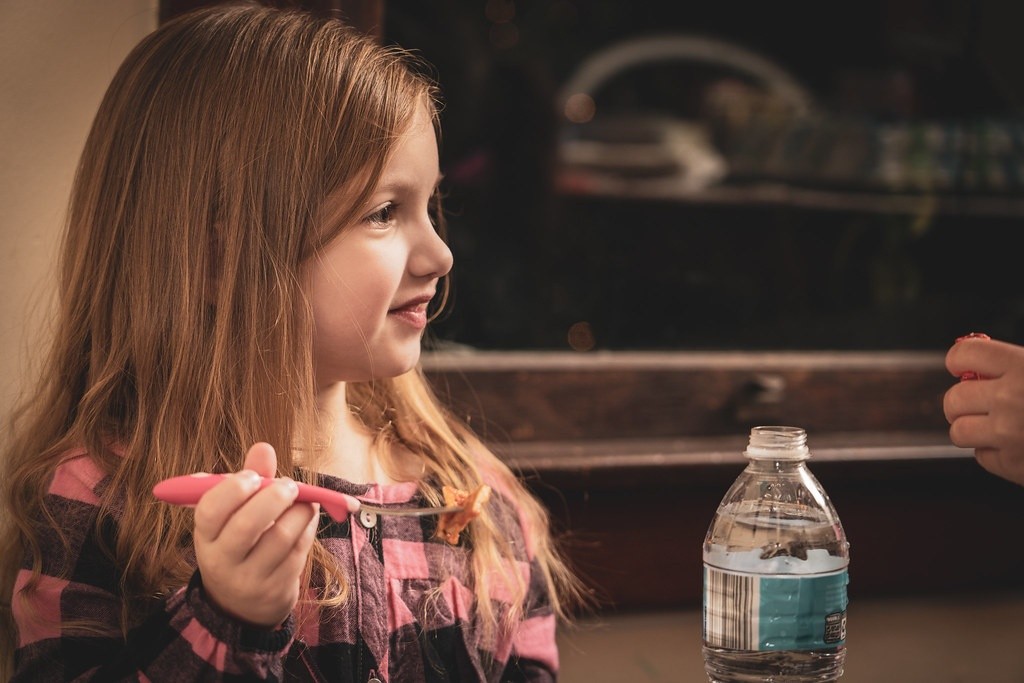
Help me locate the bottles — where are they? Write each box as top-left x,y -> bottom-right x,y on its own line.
702,425 -> 850,681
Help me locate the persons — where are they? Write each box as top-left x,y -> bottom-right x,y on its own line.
1,5 -> 596,683
942,338 -> 1024,485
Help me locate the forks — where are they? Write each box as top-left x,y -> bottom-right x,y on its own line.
152,472 -> 467,525
